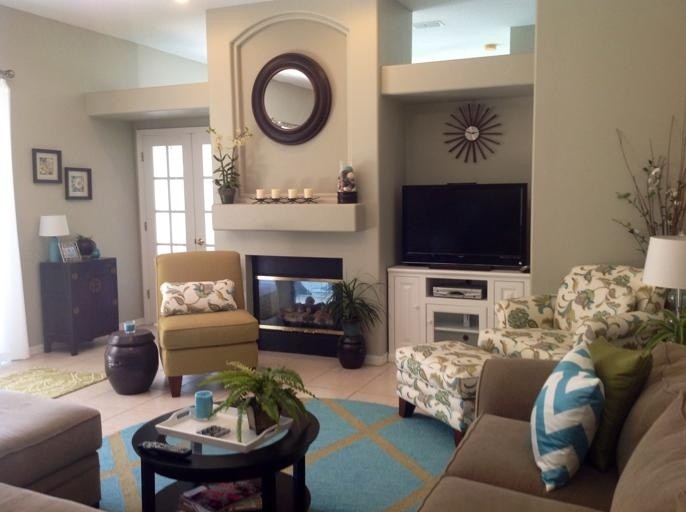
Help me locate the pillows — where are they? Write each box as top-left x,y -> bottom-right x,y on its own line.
587,337 -> 652,472
529,341 -> 606,492
159,278 -> 238,317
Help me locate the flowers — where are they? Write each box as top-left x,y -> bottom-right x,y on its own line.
205,125 -> 257,187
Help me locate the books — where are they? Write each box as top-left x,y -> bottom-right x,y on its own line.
175,479 -> 263,512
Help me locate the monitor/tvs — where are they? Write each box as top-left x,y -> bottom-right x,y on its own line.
400,183 -> 528,270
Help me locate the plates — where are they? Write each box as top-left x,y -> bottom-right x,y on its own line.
250,197 -> 271,201
265,196 -> 287,200
300,196 -> 321,201
281,195 -> 303,201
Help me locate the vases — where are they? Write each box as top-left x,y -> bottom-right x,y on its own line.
218,187 -> 236,204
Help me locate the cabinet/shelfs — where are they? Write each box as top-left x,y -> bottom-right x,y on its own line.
387,266 -> 530,365
39,258 -> 119,357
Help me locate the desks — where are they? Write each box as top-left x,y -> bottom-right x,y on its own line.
131,398 -> 320,512
104,329 -> 158,394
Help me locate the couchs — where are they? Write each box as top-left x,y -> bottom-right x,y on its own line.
154,251 -> 260,397
0,390 -> 107,512
417,340 -> 685,511
477,265 -> 676,362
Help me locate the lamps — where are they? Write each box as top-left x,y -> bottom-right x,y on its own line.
39,215 -> 70,263
642,235 -> 686,344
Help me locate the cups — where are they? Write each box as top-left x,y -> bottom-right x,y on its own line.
193,390 -> 213,421
123,320 -> 136,334
271,189 -> 281,198
303,188 -> 313,198
256,189 -> 265,198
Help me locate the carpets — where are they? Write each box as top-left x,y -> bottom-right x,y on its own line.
96,398 -> 456,510
0,366 -> 109,398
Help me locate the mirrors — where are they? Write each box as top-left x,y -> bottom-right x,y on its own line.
252,52 -> 331,144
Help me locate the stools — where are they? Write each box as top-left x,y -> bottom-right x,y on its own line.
395,341 -> 504,449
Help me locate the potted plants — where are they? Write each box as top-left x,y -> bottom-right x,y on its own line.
73,233 -> 96,255
195,361 -> 319,442
321,279 -> 386,368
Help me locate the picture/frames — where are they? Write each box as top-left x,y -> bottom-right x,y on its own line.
57,241 -> 82,262
32,148 -> 62,183
65,167 -> 92,200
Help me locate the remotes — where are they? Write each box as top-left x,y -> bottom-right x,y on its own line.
137,440 -> 192,460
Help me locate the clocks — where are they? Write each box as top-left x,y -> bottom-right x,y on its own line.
442,103 -> 502,163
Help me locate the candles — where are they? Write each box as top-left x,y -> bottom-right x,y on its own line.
256,189 -> 266,199
304,188 -> 313,199
271,189 -> 280,198
